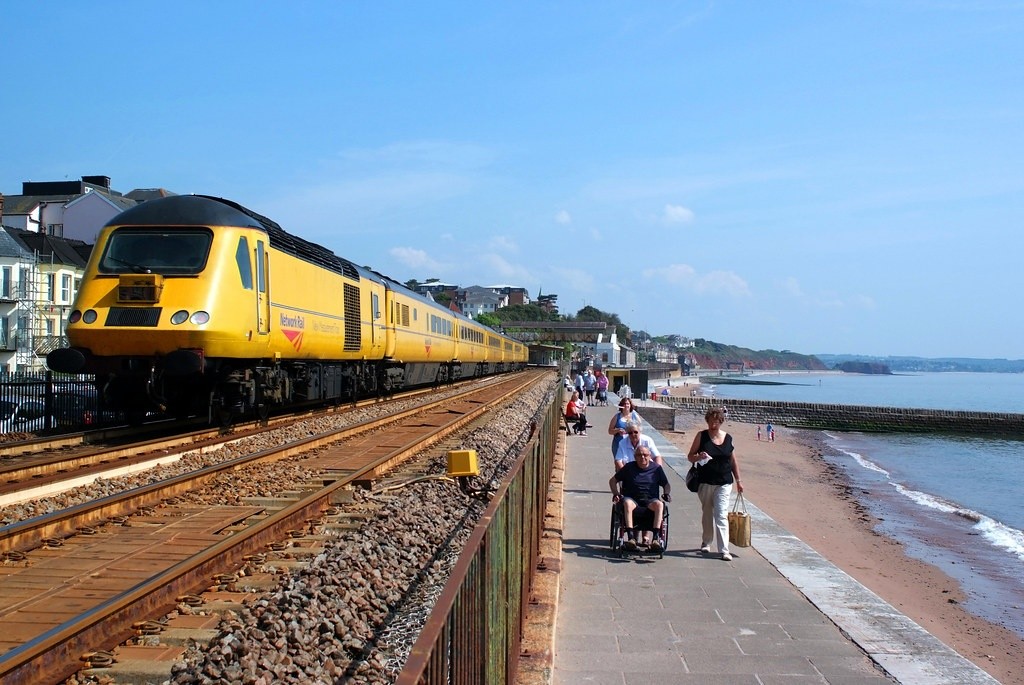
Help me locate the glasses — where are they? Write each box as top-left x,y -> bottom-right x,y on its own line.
628,431 -> 638,435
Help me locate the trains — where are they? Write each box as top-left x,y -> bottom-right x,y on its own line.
44,194 -> 529,420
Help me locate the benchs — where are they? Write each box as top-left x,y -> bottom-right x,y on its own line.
560,400 -> 580,434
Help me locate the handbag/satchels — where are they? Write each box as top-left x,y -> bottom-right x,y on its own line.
686,462 -> 702,493
727,492 -> 751,547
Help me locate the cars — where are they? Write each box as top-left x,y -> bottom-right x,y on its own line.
0,373 -> 119,433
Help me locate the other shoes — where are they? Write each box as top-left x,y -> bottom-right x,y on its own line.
580,431 -> 588,436
587,402 -> 595,406
722,553 -> 732,561
626,538 -> 637,551
701,542 -> 711,553
650,540 -> 661,550
572,425 -> 577,434
585,423 -> 593,428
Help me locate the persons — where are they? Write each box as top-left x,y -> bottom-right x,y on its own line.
609,446 -> 672,548
609,397 -> 642,460
766,422 -> 775,442
566,391 -> 592,436
688,408 -> 743,560
614,420 -> 662,546
574,370 -> 609,407
756,425 -> 762,440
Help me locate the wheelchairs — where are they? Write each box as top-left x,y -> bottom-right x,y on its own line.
610,494 -> 669,558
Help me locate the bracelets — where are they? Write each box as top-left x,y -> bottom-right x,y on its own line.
736,479 -> 742,482
